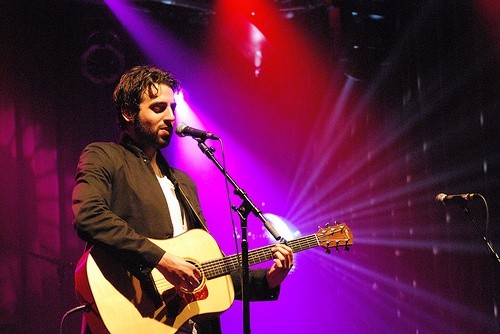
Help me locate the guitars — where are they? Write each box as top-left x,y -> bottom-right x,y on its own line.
74,219 -> 355,334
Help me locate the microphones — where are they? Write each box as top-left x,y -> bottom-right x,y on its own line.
175,122 -> 220,140
434,193 -> 479,207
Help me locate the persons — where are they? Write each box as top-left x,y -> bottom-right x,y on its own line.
71,65 -> 293,334
